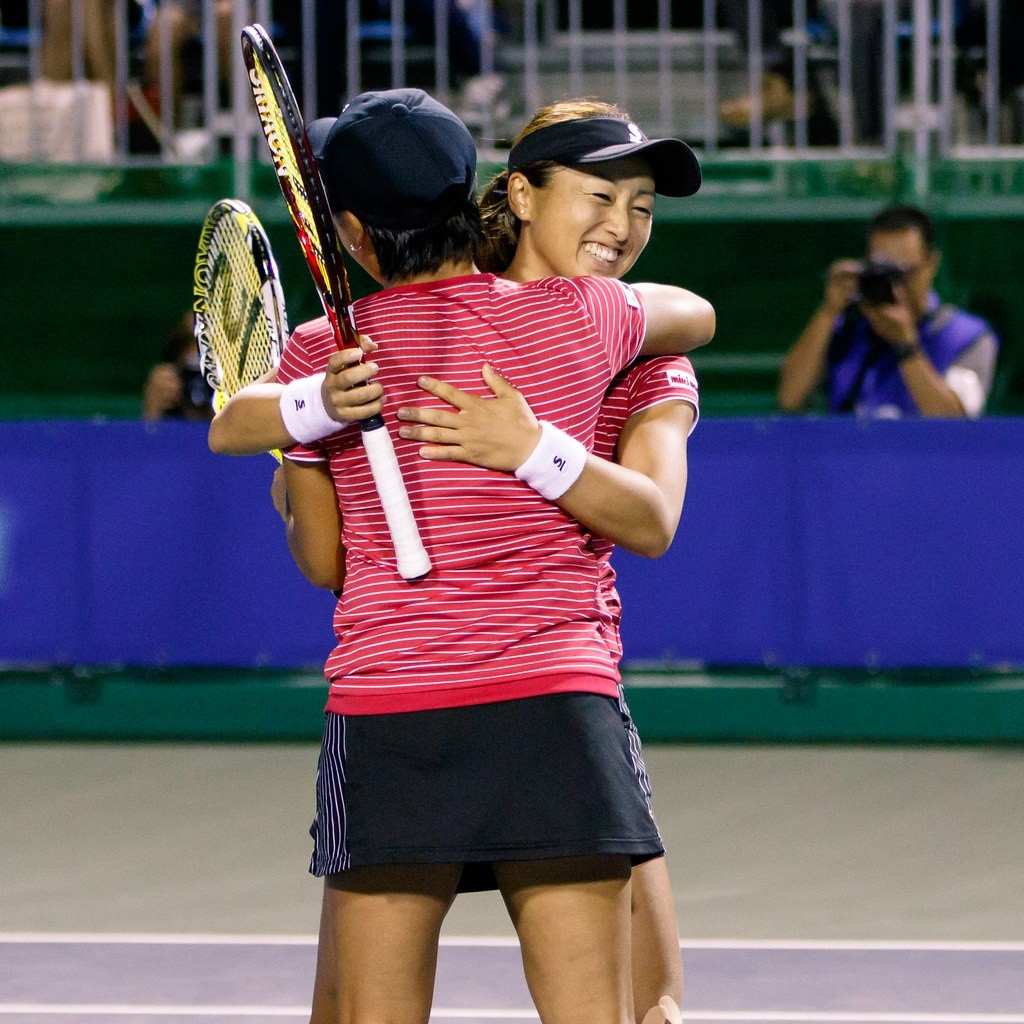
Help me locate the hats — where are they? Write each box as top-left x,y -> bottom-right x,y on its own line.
508,115 -> 702,198
305,89 -> 478,211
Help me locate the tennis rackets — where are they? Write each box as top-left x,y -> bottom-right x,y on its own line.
189,197 -> 294,465
239,20 -> 434,585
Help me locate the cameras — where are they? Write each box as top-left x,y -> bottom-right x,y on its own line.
162,362 -> 219,417
843,259 -> 910,311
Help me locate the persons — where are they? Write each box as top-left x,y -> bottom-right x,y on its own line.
36,1 -> 270,162
779,205 -> 997,421
208,86 -> 716,1024
724,60 -> 847,147
142,297 -> 217,421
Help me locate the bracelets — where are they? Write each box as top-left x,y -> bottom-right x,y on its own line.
895,344 -> 920,358
517,420 -> 585,502
280,372 -> 349,444
